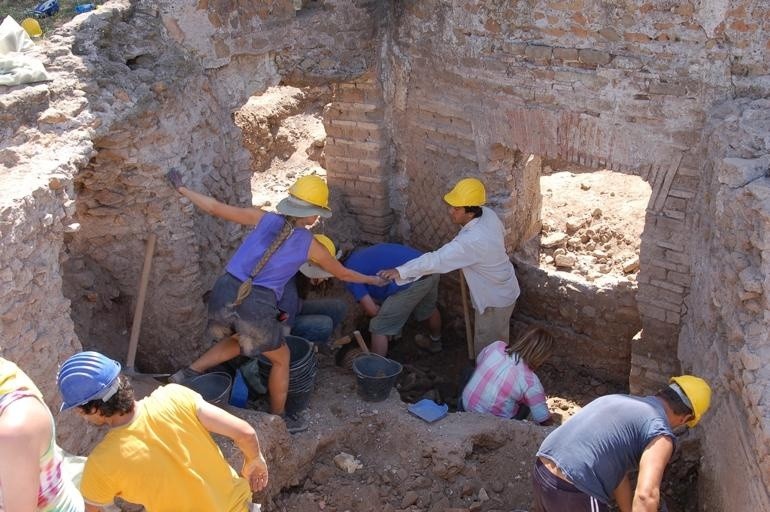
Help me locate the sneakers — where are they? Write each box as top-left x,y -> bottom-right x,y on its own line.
282,414 -> 307,432
168,366 -> 191,385
414,334 -> 442,354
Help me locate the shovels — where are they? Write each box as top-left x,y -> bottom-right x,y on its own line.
458,269 -> 476,396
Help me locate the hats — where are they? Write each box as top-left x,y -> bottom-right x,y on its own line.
299,262 -> 336,279
275,194 -> 333,218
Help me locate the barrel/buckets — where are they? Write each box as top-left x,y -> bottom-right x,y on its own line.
352,352 -> 403,402
188,371 -> 233,406
257,335 -> 315,412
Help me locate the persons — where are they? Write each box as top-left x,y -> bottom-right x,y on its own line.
334,239 -> 444,361
167,167 -> 395,434
529,374 -> 714,512
454,326 -> 557,426
0,354 -> 85,512
373,177 -> 520,365
57,350 -> 270,512
280,233 -> 349,346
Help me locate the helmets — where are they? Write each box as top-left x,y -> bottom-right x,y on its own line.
310,233 -> 342,262
443,177 -> 487,207
289,173 -> 332,210
668,374 -> 712,428
57,349 -> 121,414
